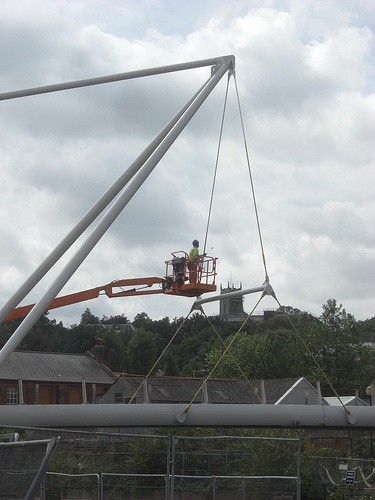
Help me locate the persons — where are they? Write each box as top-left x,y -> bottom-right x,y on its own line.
187,240 -> 203,283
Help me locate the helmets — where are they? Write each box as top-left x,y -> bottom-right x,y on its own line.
192,240 -> 199,246
